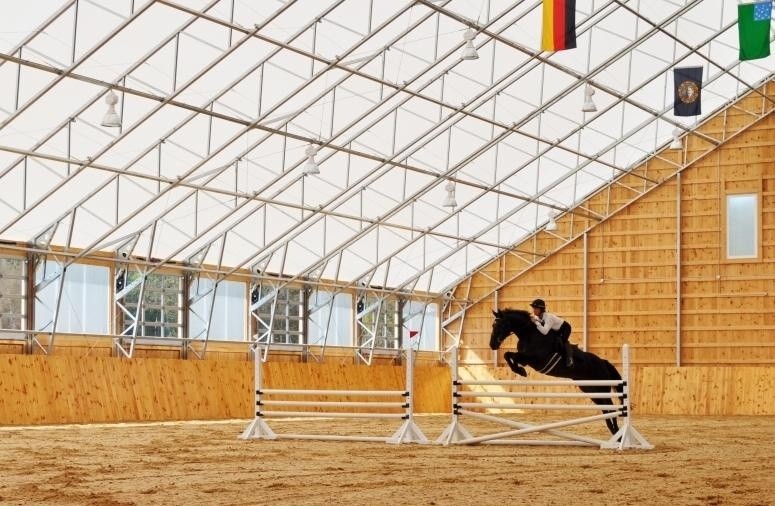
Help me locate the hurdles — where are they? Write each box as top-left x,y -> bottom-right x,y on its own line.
432,343 -> 656,452
238,348 -> 430,447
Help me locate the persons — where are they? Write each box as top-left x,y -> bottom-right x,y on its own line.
529,298 -> 574,367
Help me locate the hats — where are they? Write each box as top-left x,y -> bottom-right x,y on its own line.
529,298 -> 548,309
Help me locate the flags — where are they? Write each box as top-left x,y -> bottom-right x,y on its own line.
738,0 -> 772,60
672,64 -> 702,116
541,0 -> 577,51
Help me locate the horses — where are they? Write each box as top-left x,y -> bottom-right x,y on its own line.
487,307 -> 623,443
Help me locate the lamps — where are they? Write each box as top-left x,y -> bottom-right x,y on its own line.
546,208 -> 558,230
100,88 -> 121,128
441,180 -> 458,206
460,26 -> 480,60
580,85 -> 596,112
668,128 -> 684,150
302,144 -> 320,174
